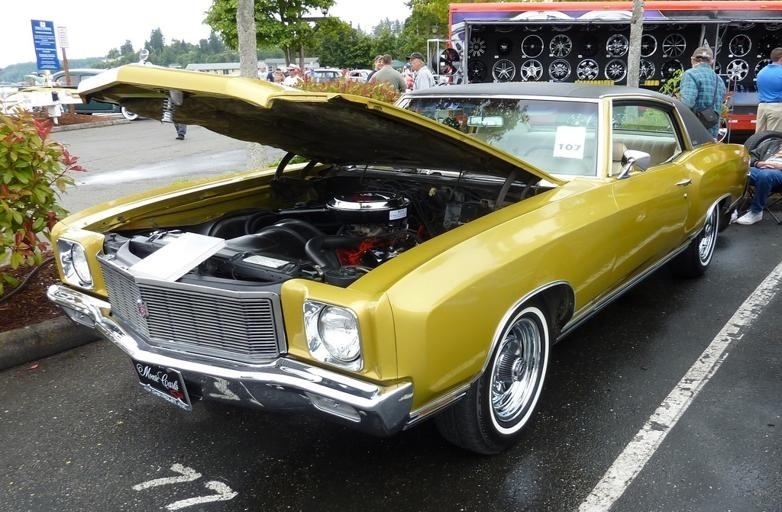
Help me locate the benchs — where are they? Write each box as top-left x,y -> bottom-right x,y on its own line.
506,128 -> 677,183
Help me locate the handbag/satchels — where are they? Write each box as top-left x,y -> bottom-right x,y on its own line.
696,107 -> 720,128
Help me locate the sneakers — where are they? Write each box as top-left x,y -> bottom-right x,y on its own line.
736,211 -> 763,224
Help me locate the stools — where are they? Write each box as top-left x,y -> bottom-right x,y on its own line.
739,182 -> 781,228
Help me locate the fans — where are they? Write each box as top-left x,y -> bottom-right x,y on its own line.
431,48 -> 461,77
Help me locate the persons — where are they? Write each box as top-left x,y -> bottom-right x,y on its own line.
173,123 -> 187,140
755,48 -> 781,133
728,140 -> 782,224
679,47 -> 725,144
257,51 -> 435,96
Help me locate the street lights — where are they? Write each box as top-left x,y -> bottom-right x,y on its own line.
321,7 -> 329,16
430,22 -> 439,53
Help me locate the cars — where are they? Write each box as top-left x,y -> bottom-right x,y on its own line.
277,66 -> 374,83
45,47 -> 753,456
42,64 -> 147,122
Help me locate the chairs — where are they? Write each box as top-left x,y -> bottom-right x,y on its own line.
591,143 -> 636,180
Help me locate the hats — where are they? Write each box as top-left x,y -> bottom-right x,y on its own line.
692,47 -> 713,61
405,52 -> 425,62
